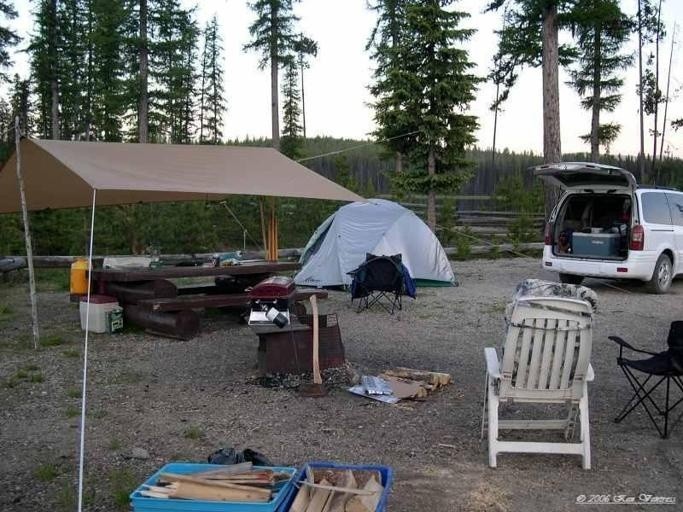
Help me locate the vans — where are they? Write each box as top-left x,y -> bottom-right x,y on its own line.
527,163 -> 683,294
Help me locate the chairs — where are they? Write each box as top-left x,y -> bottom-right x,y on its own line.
345,251 -> 417,314
477,279 -> 683,469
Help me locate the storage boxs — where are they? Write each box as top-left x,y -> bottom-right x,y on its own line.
571,230 -> 620,257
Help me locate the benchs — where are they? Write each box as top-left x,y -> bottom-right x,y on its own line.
135,289 -> 328,341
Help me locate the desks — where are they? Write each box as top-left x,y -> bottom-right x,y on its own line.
85,259 -> 306,340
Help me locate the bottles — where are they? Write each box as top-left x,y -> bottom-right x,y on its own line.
150,242 -> 160,264
255,298 -> 288,328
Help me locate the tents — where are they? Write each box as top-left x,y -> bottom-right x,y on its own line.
293,198 -> 457,289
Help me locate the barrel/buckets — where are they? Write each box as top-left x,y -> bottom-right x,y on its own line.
70,259 -> 93,295
